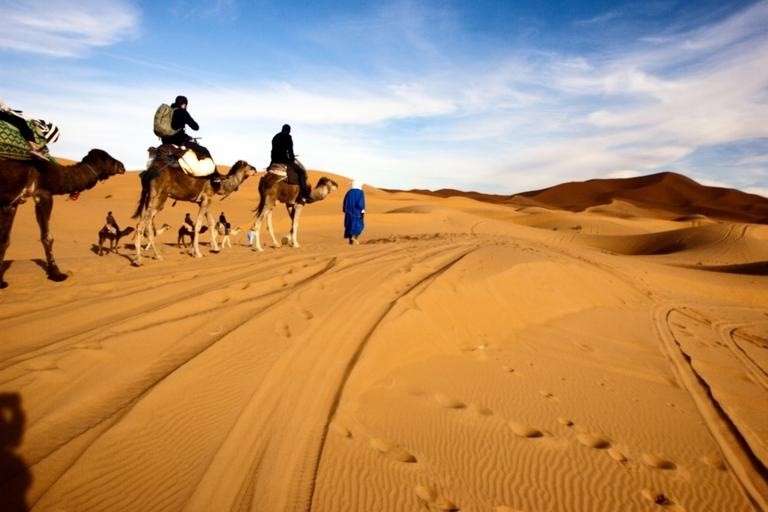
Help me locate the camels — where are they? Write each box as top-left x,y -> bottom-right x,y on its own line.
130,160 -> 258,266
94,224 -> 135,256
251,172 -> 339,251
210,222 -> 242,251
175,224 -> 209,250
140,221 -> 171,251
0,147 -> 127,292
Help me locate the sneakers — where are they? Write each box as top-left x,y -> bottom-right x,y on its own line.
213,176 -> 222,183
30,150 -> 50,162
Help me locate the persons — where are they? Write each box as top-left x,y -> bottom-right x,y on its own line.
106,211 -> 119,233
162,96 -> 226,183
266,124 -> 315,203
220,212 -> 230,229
0,99 -> 44,152
342,179 -> 366,245
247,226 -> 256,246
185,213 -> 194,230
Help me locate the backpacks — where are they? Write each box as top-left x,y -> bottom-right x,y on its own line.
153,104 -> 182,136
